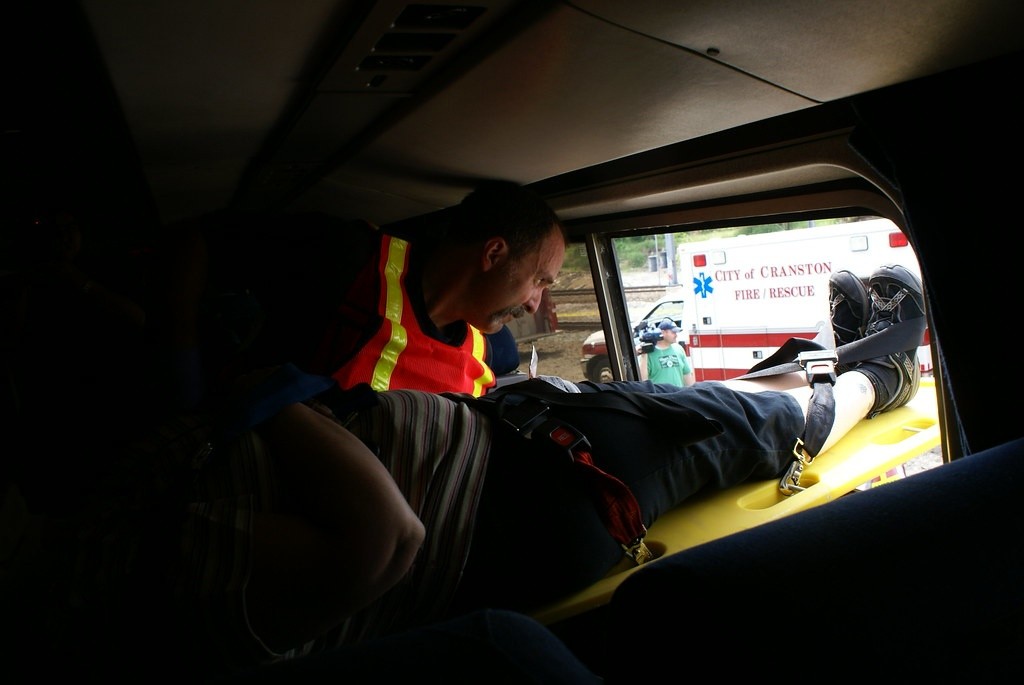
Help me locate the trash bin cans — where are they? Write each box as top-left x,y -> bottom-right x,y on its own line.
648,256 -> 657,272
660,251 -> 667,269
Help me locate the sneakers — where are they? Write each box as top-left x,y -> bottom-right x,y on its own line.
855,264 -> 926,420
829,268 -> 869,374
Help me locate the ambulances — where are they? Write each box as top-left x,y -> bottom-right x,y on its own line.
579,217 -> 934,393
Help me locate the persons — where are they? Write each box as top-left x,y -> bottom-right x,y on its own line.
639,318 -> 694,388
0,263 -> 927,685
229,178 -> 569,422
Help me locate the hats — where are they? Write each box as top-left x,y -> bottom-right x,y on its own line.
658,320 -> 683,333
